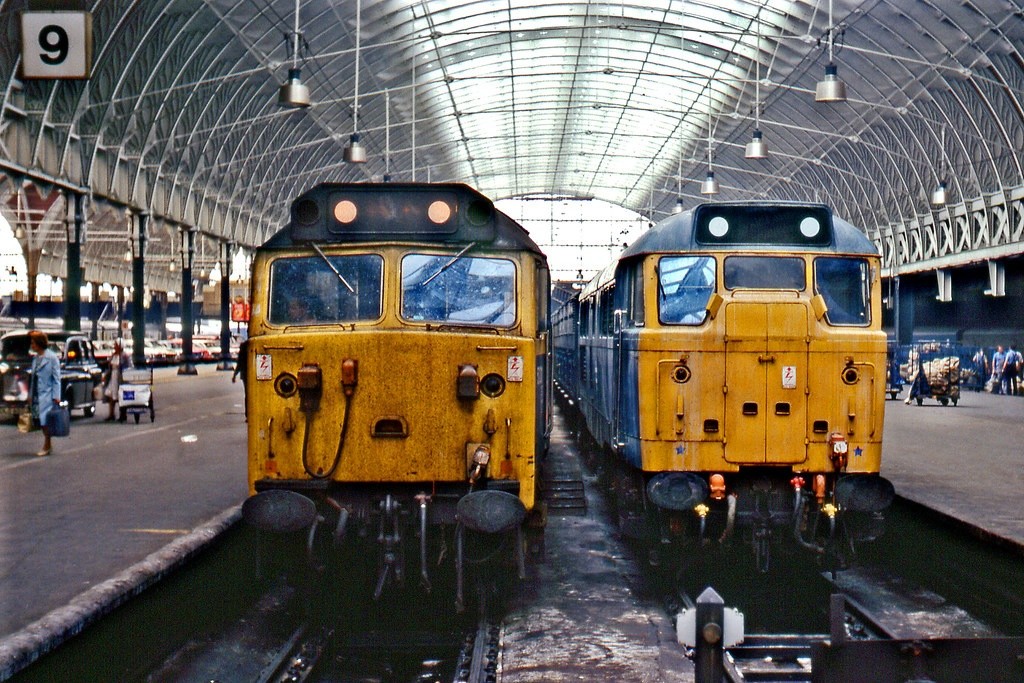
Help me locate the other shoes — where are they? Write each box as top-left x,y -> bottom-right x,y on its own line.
118,415 -> 128,422
37,447 -> 51,456
104,415 -> 116,422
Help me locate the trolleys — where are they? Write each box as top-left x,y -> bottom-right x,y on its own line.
118,366 -> 156,424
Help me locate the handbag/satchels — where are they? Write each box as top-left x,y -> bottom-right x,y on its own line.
46,402 -> 70,437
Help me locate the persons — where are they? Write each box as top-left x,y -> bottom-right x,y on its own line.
992,346 -> 1006,395
101,337 -> 133,422
285,297 -> 319,324
232,328 -> 248,423
1001,346 -> 1019,396
26,331 -> 61,456
973,349 -> 990,392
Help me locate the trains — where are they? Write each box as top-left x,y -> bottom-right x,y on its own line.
550,200 -> 895,582
240,181 -> 550,612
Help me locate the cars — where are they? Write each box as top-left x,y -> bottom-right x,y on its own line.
0,327 -> 101,422
91,333 -> 247,366
